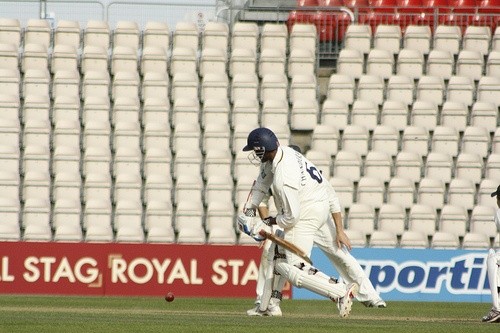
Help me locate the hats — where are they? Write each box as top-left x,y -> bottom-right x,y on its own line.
243,128 -> 278,153
490,185 -> 500,198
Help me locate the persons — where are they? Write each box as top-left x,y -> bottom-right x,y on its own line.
481,185 -> 500,323
238,128 -> 386,319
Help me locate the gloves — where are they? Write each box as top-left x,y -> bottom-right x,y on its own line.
238,214 -> 258,235
250,222 -> 272,242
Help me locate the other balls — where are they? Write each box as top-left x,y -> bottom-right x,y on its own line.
165,294 -> 175,301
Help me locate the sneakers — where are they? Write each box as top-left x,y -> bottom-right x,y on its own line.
336,284 -> 358,319
364,300 -> 386,308
247,304 -> 283,317
482,310 -> 499,323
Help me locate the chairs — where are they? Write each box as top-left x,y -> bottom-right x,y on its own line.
0,0 -> 500,247
